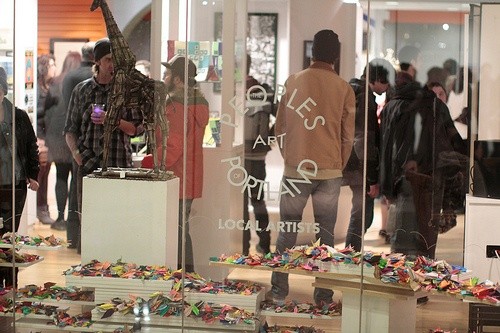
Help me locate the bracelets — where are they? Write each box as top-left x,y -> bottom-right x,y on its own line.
72,149 -> 80,159
117,120 -> 121,128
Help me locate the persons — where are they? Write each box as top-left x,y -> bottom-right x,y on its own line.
263,29 -> 356,305
342,46 -> 500,307
37,37 -> 144,254
0,68 -> 39,290
243,55 -> 281,257
140,54 -> 210,273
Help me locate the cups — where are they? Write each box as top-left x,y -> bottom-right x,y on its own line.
92,104 -> 105,124
